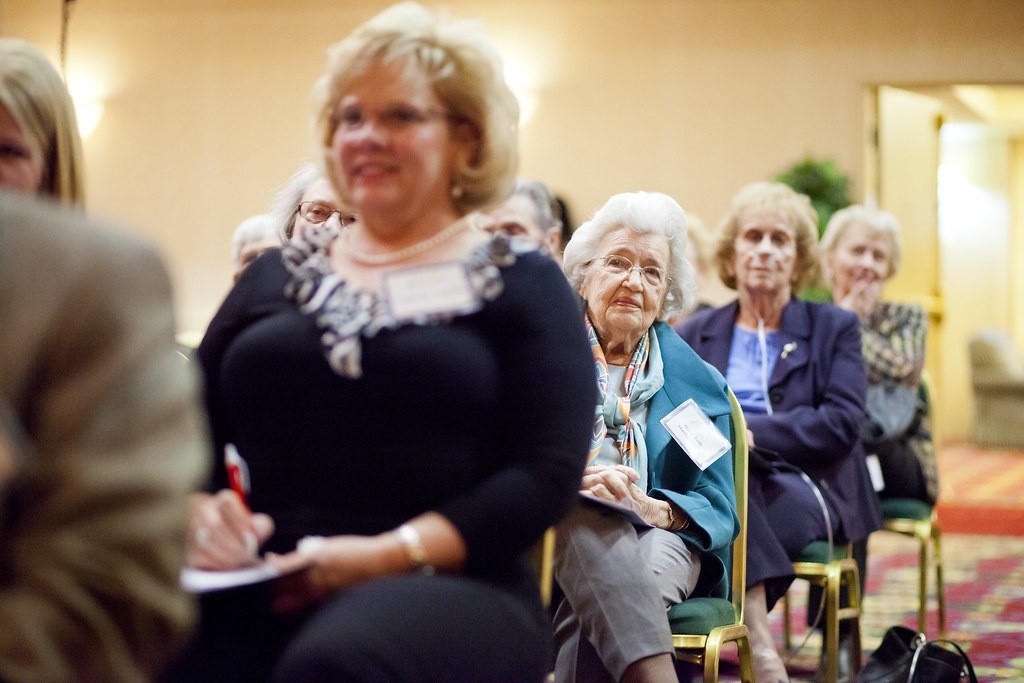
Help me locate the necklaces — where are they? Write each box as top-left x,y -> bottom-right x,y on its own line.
347,216 -> 473,264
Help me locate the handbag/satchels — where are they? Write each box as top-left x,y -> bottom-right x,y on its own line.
856,623 -> 976,683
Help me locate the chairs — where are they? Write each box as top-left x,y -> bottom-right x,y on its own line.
785,539 -> 863,683
869,377 -> 945,639
670,384 -> 754,682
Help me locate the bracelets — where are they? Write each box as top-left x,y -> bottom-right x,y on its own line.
397,525 -> 428,572
666,502 -> 676,529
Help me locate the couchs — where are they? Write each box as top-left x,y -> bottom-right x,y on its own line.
970,333 -> 1024,448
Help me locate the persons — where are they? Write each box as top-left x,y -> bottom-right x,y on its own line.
553,192 -> 740,683
473,176 -> 572,267
0,192 -> 203,683
668,215 -> 713,329
232,163 -> 357,288
675,181 -> 883,683
0,36 -> 87,217
791,205 -> 939,657
151,0 -> 599,683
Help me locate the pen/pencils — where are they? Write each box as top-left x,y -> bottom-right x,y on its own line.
220,441 -> 262,563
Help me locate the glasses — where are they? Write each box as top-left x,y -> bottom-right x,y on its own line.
589,255 -> 673,284
294,201 -> 360,227
